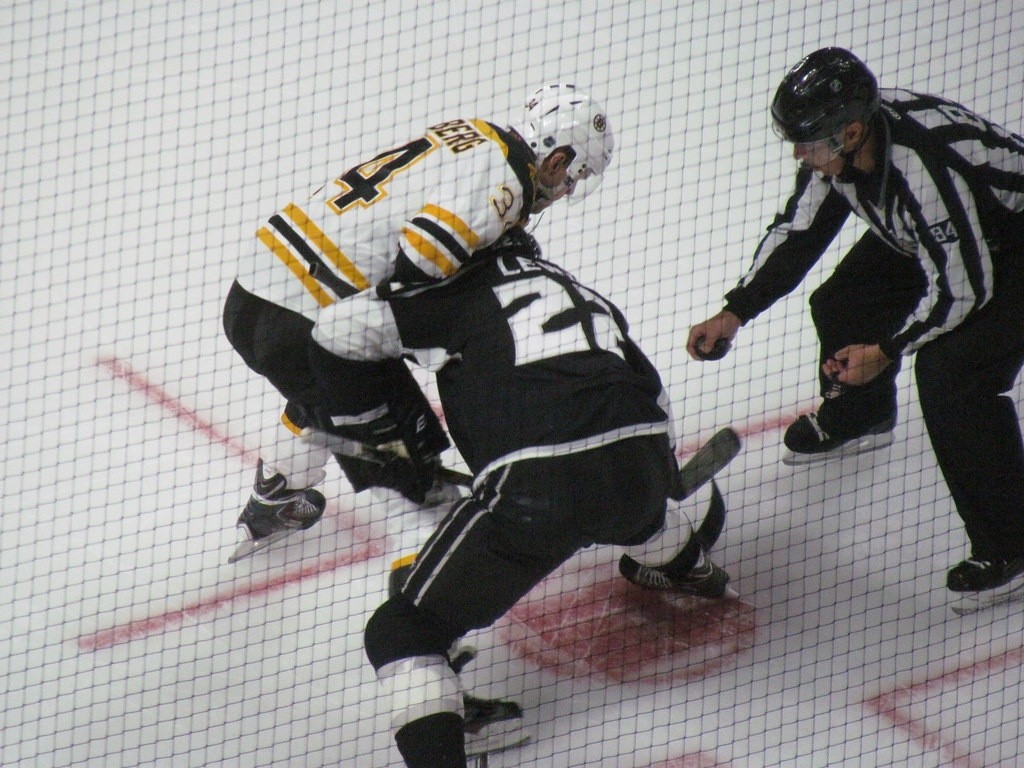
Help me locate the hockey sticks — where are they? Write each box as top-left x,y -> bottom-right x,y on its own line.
303,429 -> 747,505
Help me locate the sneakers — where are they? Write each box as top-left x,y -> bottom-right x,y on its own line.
944,545 -> 1024,616
616,548 -> 741,602
782,353 -> 901,461
418,452 -> 463,506
447,646 -> 533,754
229,457 -> 327,563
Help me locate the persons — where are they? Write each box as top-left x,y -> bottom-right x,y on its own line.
687,47 -> 1024,615
309,228 -> 747,768
222,81 -> 615,562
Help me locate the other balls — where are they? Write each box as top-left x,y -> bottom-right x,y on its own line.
693,334 -> 730,362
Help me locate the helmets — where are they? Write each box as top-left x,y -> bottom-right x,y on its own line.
771,45 -> 881,143
508,82 -> 614,198
487,224 -> 544,259
